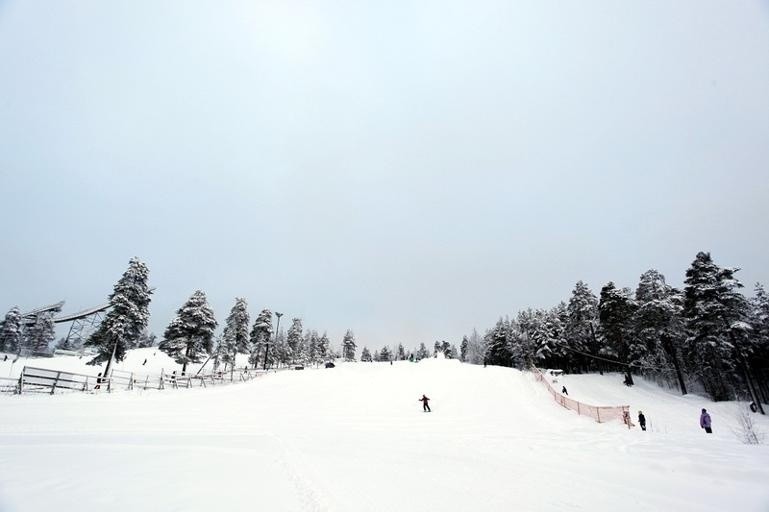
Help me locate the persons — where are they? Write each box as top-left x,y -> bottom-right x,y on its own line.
562,383 -> 569,395
638,411 -> 646,431
700,408 -> 712,433
414,396 -> 431,412
